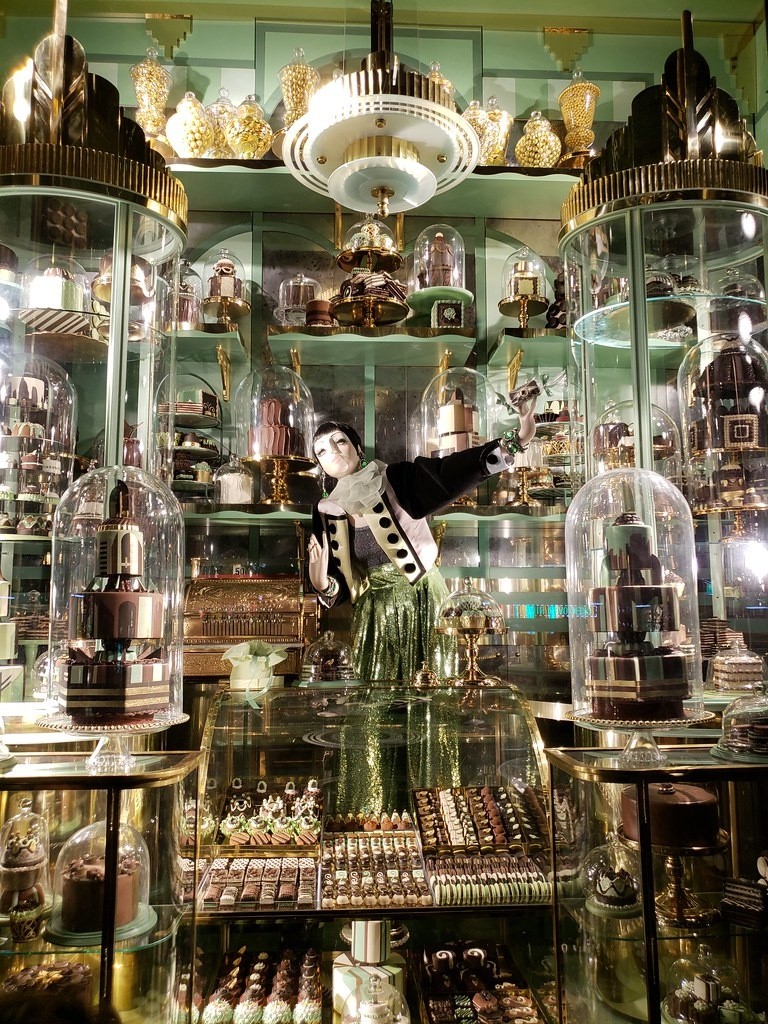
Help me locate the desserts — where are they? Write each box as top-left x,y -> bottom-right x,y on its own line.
1,34 -> 768,1024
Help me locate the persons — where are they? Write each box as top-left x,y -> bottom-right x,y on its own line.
308,395 -> 537,813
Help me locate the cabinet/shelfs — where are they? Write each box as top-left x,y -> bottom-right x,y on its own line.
0,0 -> 768,1024
150,154 -> 716,534
197,686 -> 559,1023
540,746 -> 768,1024
0,750 -> 206,1024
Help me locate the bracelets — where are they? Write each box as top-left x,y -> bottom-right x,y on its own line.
500,429 -> 528,457
